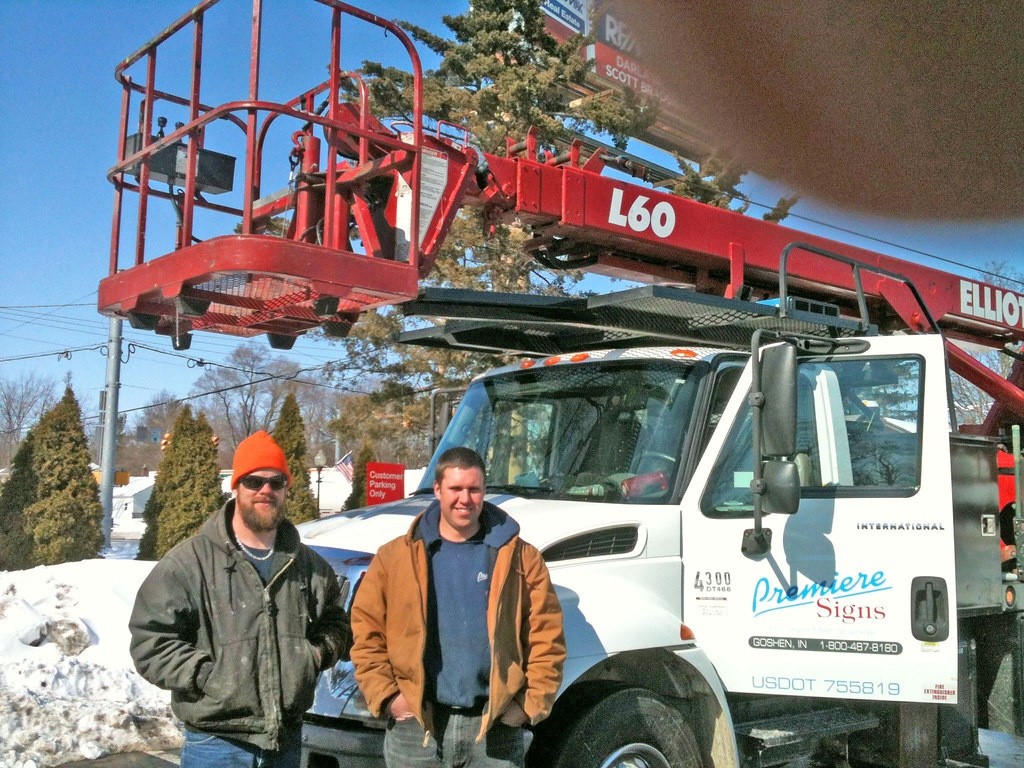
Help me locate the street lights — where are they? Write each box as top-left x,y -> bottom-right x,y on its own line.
314,449 -> 327,519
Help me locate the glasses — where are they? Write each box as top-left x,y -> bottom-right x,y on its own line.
239,474 -> 287,491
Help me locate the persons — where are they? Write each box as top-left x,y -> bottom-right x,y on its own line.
350,447 -> 567,768
127,430 -> 350,768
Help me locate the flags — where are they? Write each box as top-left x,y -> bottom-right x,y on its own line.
337,454 -> 354,483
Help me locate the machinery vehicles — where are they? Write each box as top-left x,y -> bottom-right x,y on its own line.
95,0 -> 1024,768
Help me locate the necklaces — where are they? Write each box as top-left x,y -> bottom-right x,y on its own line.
233,533 -> 274,561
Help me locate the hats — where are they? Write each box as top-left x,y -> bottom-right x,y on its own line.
231,430 -> 292,490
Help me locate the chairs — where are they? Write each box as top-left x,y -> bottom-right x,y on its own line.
578,420 -> 643,478
845,420 -> 890,486
